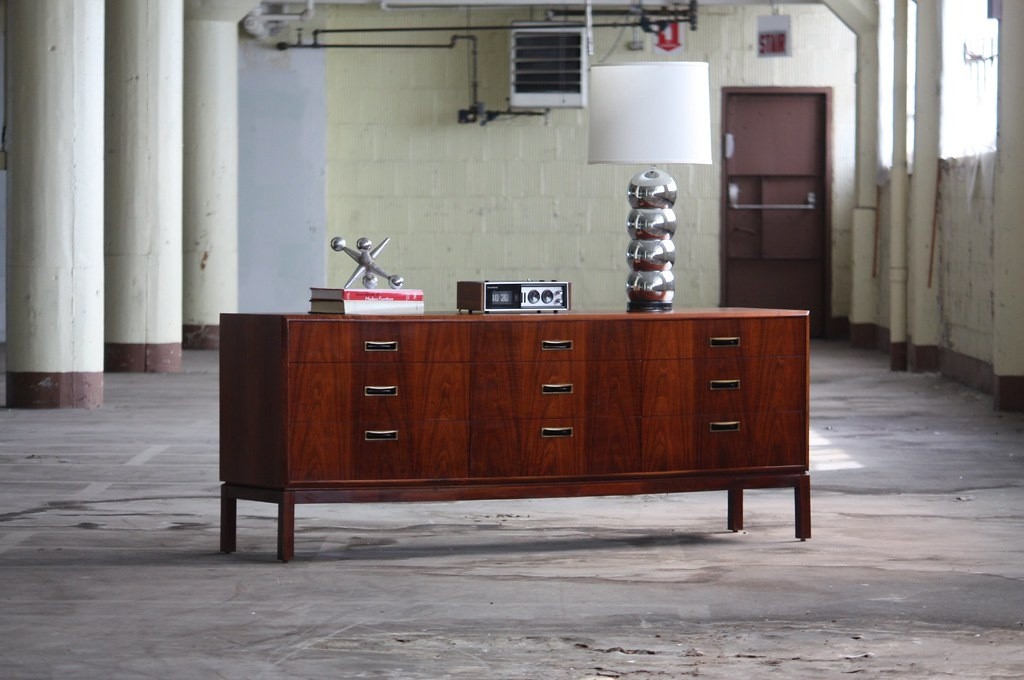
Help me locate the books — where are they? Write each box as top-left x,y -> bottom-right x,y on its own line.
308,286 -> 426,316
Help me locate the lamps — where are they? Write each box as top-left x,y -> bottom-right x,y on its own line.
587,59 -> 713,314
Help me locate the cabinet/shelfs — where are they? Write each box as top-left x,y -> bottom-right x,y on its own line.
215,309 -> 814,562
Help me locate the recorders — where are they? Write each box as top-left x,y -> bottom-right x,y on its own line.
456,280 -> 572,313
